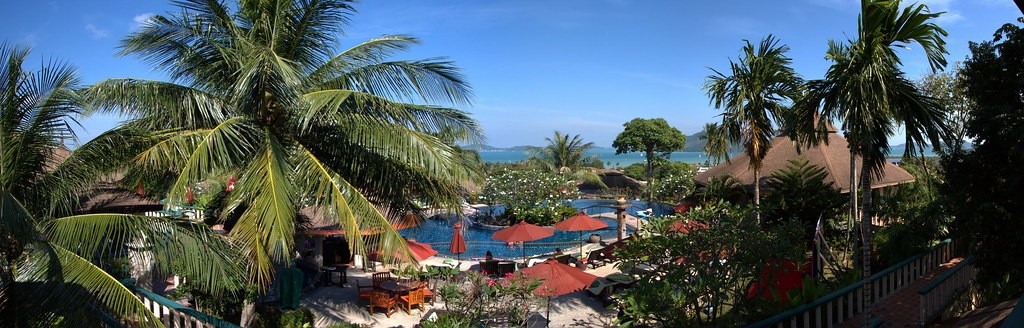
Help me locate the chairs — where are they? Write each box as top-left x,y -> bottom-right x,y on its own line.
357,272 -> 438,318
426,238 -> 628,280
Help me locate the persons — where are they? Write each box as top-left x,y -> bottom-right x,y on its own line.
486,251 -> 493,261
553,248 -> 562,259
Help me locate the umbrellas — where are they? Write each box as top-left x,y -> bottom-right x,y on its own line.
449,221 -> 467,267
553,212 -> 608,259
367,241 -> 438,283
515,260 -> 598,328
492,220 -> 555,269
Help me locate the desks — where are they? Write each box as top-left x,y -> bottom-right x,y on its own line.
324,264 -> 348,287
379,279 -> 420,304
